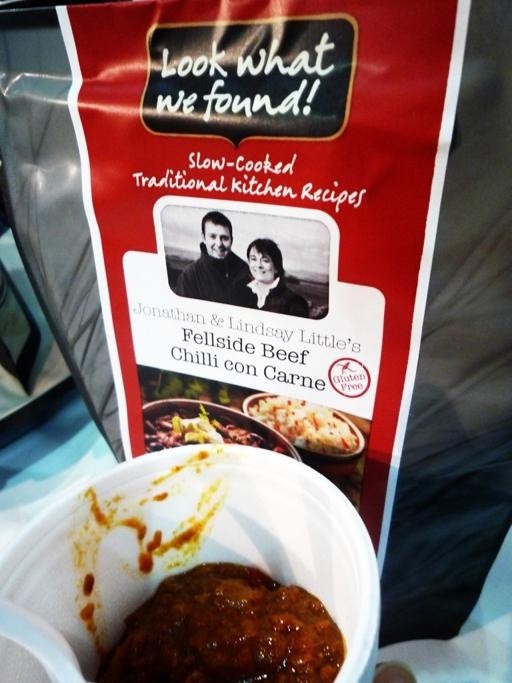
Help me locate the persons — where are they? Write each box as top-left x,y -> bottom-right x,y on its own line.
233,236 -> 308,316
177,208 -> 252,306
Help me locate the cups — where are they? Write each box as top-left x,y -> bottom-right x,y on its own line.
0,445 -> 384,682
